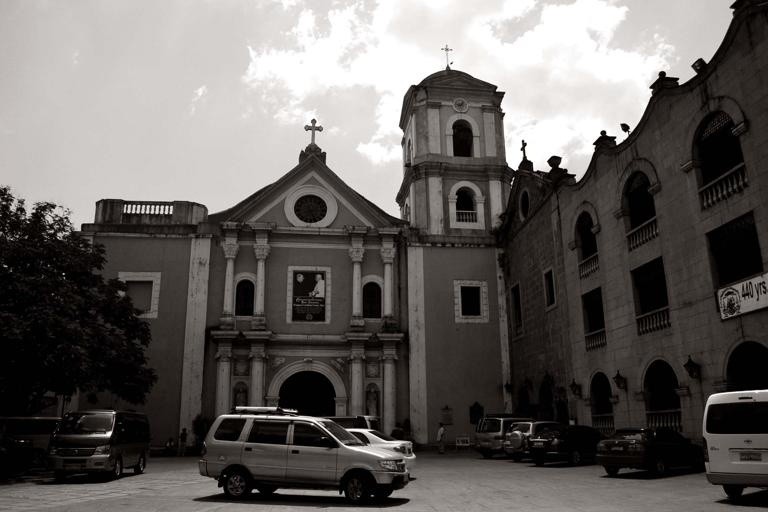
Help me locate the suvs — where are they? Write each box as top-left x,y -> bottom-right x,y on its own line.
501,420 -> 563,463
199,404 -> 417,503
322,415 -> 381,431
475,413 -> 538,458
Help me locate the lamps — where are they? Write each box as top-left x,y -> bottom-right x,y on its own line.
542,370 -> 554,386
682,353 -> 700,379
613,370 -> 626,389
504,380 -> 513,394
568,378 -> 582,400
520,376 -> 532,390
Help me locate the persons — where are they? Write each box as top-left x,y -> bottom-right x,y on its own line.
391,422 -> 404,439
313,274 -> 325,297
436,423 -> 444,453
180,429 -> 187,457
294,274 -> 305,296
367,388 -> 377,415
236,387 -> 246,406
165,438 -> 175,449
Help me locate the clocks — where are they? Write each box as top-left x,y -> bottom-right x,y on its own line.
453,98 -> 468,113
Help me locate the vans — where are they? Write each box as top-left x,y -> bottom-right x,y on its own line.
47,409 -> 152,480
2,415 -> 61,464
702,388 -> 768,498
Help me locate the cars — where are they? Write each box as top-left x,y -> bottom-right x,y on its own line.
0,432 -> 32,485
345,427 -> 417,478
529,426 -> 608,466
595,427 -> 703,477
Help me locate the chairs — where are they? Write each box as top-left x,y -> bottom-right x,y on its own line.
455,437 -> 472,453
